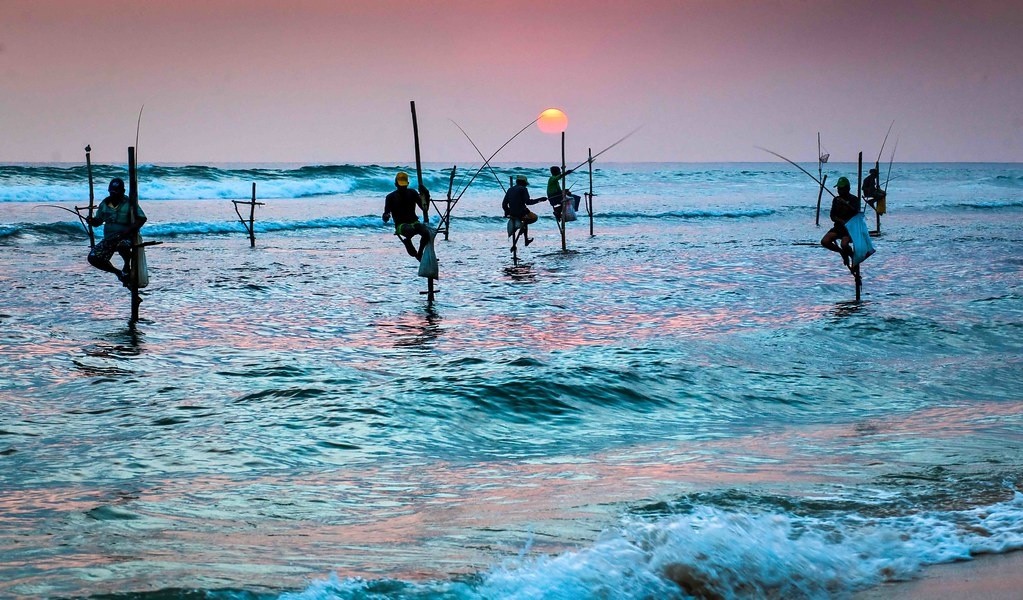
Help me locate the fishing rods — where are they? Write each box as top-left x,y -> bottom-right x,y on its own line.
871,119 -> 904,191
31,203 -> 87,220
134,103 -> 146,229
433,115 -> 544,240
546,123 -> 642,202
750,143 -> 837,200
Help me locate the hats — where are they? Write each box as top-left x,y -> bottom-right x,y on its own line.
869,169 -> 880,174
396,173 -> 408,186
517,175 -> 530,185
108,178 -> 124,193
834,177 -> 850,188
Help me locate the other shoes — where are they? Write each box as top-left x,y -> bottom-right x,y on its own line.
525,237 -> 534,246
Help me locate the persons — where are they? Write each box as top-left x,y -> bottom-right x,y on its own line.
502,175 -> 547,246
382,172 -> 431,262
547,166 -> 581,222
862,168 -> 886,208
85,178 -> 147,282
821,177 -> 859,265
